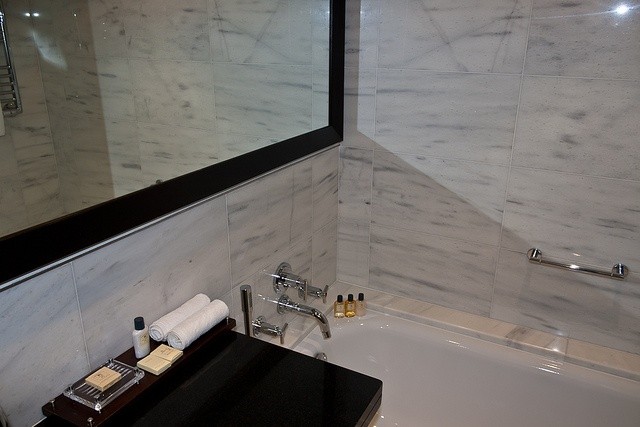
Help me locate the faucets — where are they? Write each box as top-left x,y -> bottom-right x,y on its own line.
279,294 -> 332,340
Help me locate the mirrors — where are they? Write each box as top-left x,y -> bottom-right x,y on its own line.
0,0 -> 346,295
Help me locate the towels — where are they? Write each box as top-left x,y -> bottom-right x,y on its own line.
149,293 -> 211,341
167,298 -> 229,349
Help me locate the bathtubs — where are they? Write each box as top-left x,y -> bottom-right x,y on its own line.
282,298 -> 640,427
